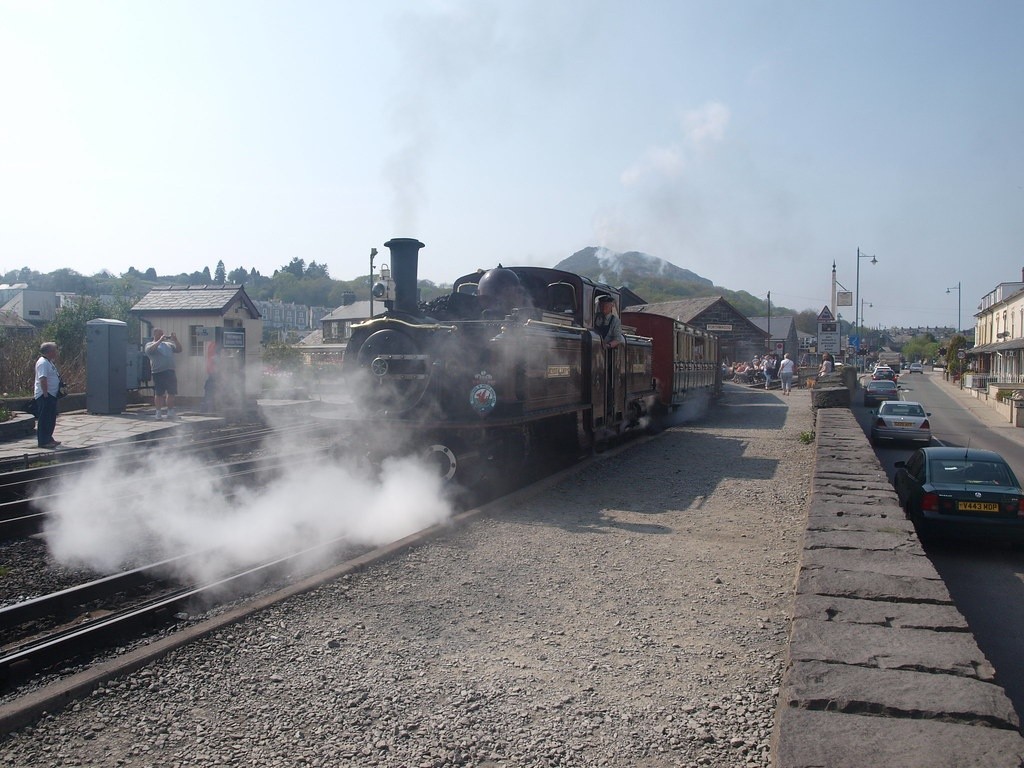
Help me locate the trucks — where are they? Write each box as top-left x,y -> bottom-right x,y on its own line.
879,353 -> 902,373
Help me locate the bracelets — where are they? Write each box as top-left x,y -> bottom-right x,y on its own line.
607,342 -> 611,347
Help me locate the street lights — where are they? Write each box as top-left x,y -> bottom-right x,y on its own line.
369,246 -> 380,319
862,302 -> 873,327
855,254 -> 879,333
945,287 -> 961,332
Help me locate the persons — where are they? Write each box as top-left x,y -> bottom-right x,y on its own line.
723,354 -> 779,389
820,351 -> 834,376
145,329 -> 182,420
35,342 -> 61,447
595,295 -> 622,348
778,353 -> 795,396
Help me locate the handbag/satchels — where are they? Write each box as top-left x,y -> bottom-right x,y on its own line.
57,382 -> 67,399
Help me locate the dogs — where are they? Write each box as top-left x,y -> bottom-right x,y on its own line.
804,377 -> 817,390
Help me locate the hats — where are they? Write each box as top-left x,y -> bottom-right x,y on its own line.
599,296 -> 615,303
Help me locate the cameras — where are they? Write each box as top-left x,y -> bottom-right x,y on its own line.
166,335 -> 172,339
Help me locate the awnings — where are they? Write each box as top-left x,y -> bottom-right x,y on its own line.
966,337 -> 1024,352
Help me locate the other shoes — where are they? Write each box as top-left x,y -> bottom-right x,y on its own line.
40,443 -> 55,448
155,411 -> 163,421
51,439 -> 61,445
168,413 -> 181,419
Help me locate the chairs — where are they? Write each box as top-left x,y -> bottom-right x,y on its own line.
972,464 -> 985,472
886,407 -> 893,413
908,408 -> 918,416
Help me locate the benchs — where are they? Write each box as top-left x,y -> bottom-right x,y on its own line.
738,370 -> 756,384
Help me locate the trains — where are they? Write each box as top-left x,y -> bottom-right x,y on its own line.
313,238 -> 720,501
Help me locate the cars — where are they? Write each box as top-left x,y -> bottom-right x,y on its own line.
870,401 -> 932,447
909,363 -> 924,374
871,367 -> 900,386
863,380 -> 901,407
894,437 -> 1024,551
